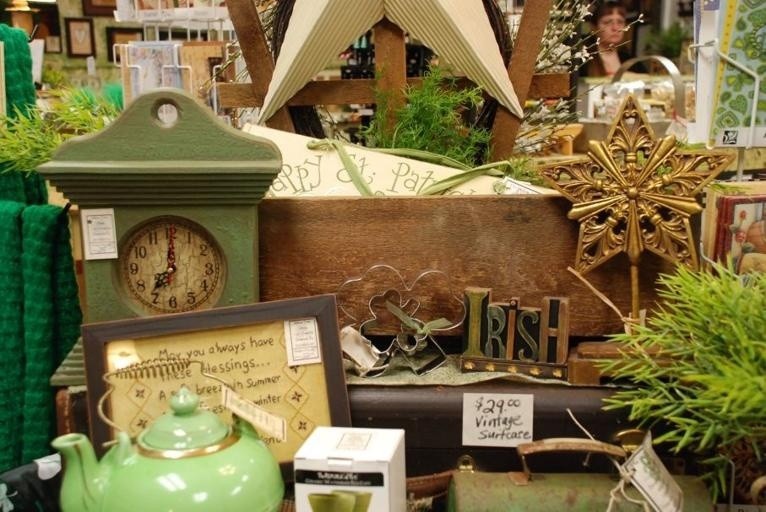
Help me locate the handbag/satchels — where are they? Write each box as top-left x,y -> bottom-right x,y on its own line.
448,436 -> 713,511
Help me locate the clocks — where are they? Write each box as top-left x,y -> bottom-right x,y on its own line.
29,95 -> 280,383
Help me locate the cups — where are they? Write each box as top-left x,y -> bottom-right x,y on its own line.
306,489 -> 371,512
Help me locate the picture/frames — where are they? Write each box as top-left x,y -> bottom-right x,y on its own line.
77,286 -> 355,491
63,0 -> 143,63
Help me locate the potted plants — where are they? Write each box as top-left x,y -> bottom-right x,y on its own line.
587,265 -> 766,507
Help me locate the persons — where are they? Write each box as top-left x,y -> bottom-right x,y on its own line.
572,3 -> 646,96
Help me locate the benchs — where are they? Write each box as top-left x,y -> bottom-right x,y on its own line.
247,188 -> 701,484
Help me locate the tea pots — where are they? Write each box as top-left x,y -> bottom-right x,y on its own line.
48,385 -> 287,512
